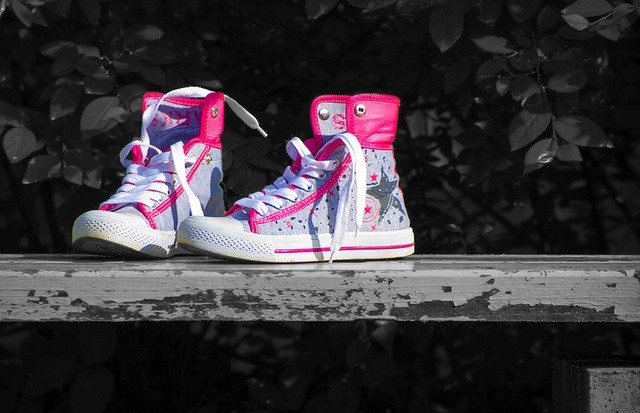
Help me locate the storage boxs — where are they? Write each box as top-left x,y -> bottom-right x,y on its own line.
1,253 -> 636,413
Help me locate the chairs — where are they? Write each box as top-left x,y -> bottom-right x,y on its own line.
177,95 -> 414,264
72,87 -> 267,258
